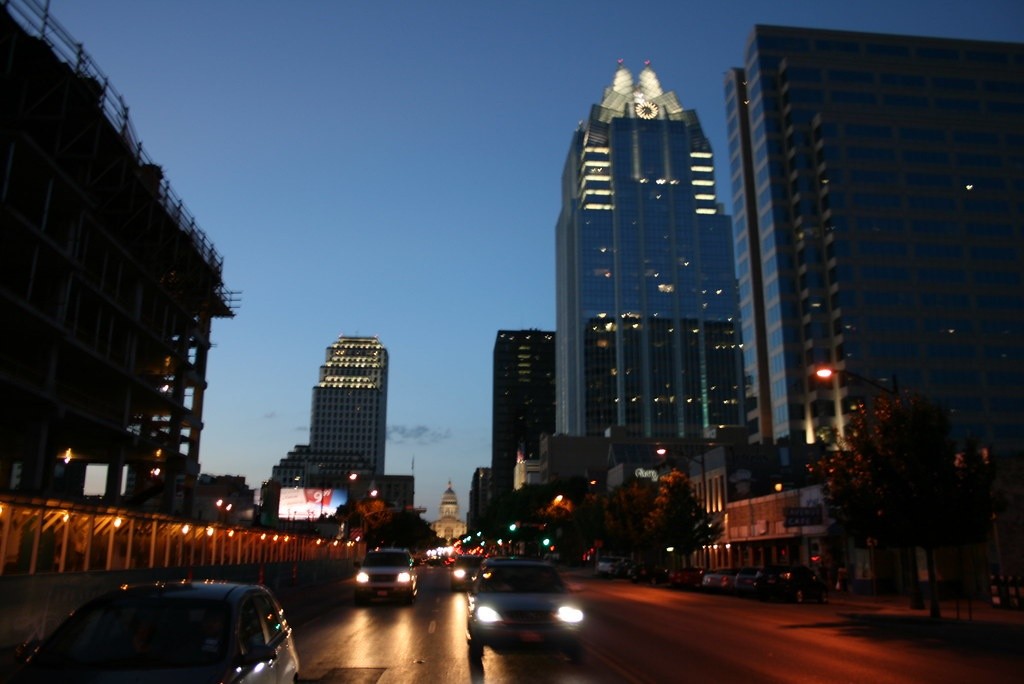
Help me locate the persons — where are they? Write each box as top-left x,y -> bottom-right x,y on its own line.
839,565 -> 849,592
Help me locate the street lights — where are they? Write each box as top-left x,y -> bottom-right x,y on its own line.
816,366 -> 925,610
343,472 -> 358,541
655,443 -> 711,572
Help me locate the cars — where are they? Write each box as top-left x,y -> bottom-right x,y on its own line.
466,557 -> 587,664
596,554 -> 831,605
7,579 -> 299,684
449,554 -> 485,591
352,548 -> 420,605
